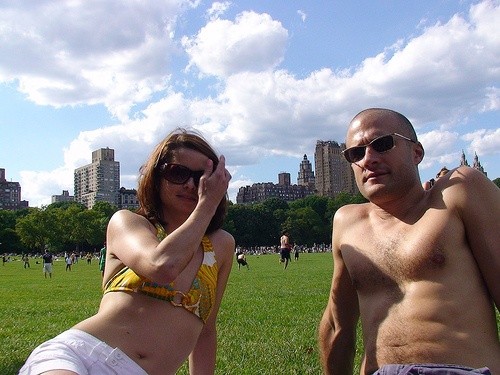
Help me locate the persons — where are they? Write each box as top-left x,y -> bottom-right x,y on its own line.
18,126 -> 236,375
0,229 -> 332,279
317,107 -> 500,375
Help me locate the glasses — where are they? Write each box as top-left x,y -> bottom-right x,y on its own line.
340,132 -> 418,164
158,162 -> 206,189
